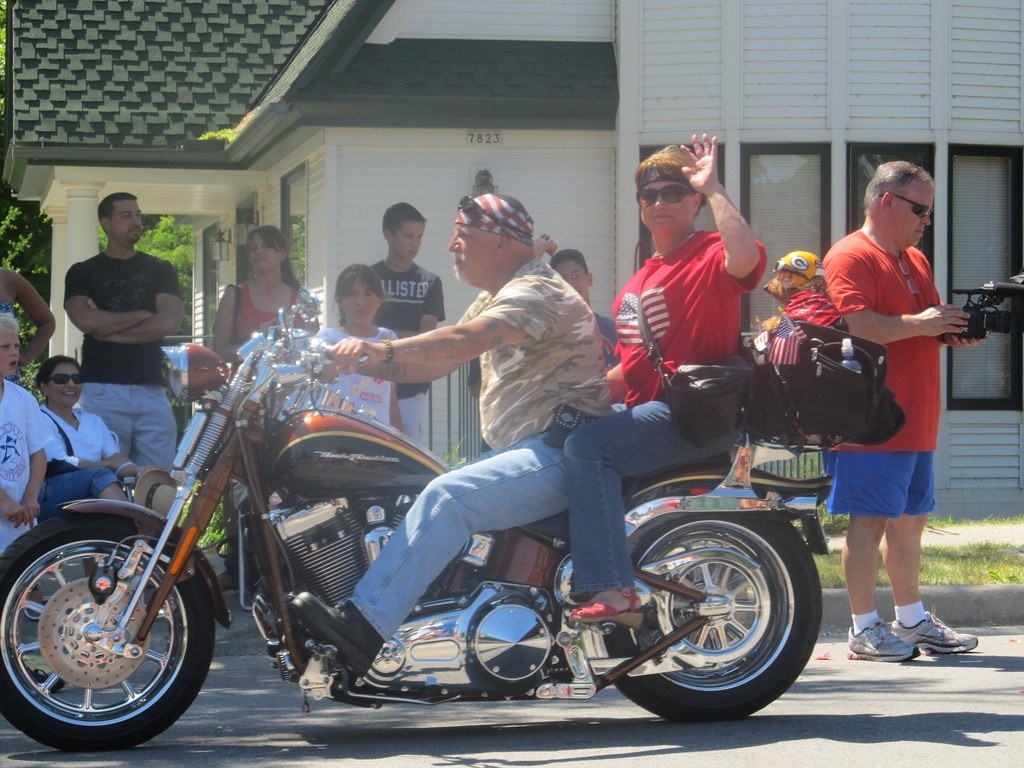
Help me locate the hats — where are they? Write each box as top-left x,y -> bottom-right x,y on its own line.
455,193 -> 534,247
133,469 -> 183,526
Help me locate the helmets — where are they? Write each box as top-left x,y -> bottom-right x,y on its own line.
776,251 -> 824,288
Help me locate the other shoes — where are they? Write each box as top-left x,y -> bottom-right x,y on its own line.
24,584 -> 44,620
31,669 -> 65,691
217,571 -> 232,590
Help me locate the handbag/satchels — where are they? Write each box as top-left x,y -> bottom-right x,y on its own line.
39,409 -> 80,478
793,320 -> 907,445
670,346 -> 759,448
745,361 -> 800,438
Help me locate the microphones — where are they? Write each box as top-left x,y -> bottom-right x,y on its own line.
995,282 -> 1024,297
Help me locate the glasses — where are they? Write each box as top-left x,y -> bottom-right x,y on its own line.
49,373 -> 83,384
897,257 -> 918,293
773,261 -> 809,281
880,193 -> 933,218
561,269 -> 586,286
458,196 -> 526,234
636,184 -> 695,207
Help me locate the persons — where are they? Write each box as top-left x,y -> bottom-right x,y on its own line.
210,226 -> 321,376
294,193 -> 611,674
63,193 -> 185,621
0,268 -> 55,385
311,264 -> 406,430
549,248 -> 621,372
0,312 -> 53,554
371,202 -> 445,449
32,355 -> 166,524
819,160 -> 987,662
564,133 -> 769,627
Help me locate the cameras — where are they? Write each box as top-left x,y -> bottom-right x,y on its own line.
925,289 -> 1012,340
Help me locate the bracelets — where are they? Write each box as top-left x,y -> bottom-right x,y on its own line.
378,338 -> 393,363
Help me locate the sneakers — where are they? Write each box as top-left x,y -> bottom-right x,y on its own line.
891,603 -> 978,653
291,592 -> 384,677
848,619 -> 921,662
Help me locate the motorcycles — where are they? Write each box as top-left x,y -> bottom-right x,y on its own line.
0,275 -> 909,756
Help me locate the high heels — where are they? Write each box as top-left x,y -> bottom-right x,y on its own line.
571,587 -> 644,629
